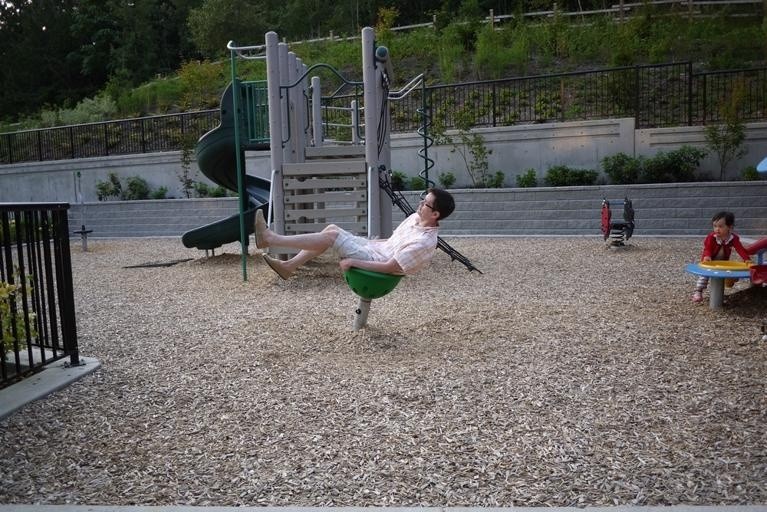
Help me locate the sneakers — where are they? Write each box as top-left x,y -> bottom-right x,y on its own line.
256,209 -> 271,248
263,253 -> 293,281
692,292 -> 703,302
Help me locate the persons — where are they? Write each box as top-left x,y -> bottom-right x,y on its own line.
692,210 -> 753,304
253,188 -> 455,280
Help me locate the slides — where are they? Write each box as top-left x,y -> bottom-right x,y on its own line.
182,79 -> 274,250
